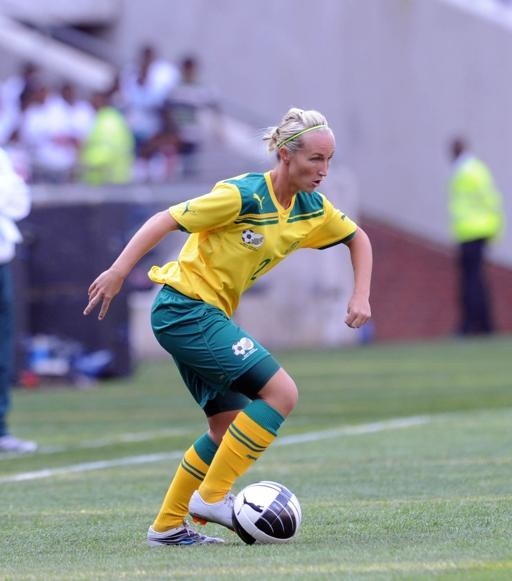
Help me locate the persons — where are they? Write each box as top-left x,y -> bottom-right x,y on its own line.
0,146 -> 39,455
0,41 -> 229,184
440,134 -> 503,336
84,106 -> 373,550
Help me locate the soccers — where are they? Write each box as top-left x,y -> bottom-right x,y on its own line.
242,229 -> 254,243
232,343 -> 245,356
231,480 -> 304,545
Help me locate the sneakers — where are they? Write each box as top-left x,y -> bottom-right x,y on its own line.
1,436 -> 37,452
147,519 -> 225,546
188,489 -> 237,534
